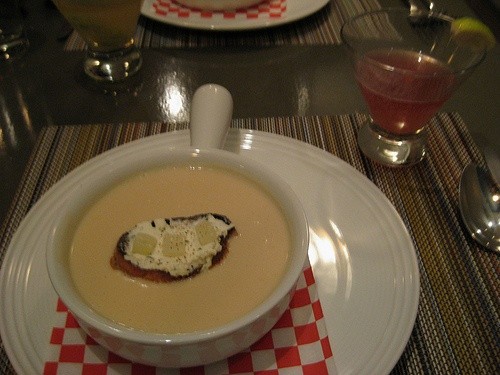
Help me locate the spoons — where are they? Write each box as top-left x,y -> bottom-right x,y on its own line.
459,162 -> 500,254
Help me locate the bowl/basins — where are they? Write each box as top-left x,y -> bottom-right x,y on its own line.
45,84 -> 309,367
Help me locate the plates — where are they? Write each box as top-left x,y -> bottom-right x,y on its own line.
1,128 -> 420,374
141,0 -> 329,30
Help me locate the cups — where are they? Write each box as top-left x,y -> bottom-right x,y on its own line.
53,0 -> 146,82
340,7 -> 488,167
